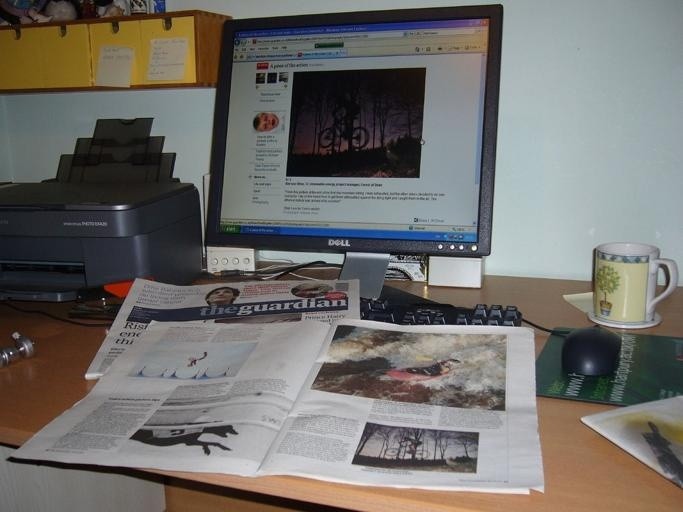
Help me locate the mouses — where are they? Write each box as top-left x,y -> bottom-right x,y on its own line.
561,321 -> 624,375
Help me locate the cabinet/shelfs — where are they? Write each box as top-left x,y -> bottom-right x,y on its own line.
0,9 -> 233,95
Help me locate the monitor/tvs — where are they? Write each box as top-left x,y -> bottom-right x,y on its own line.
204,2 -> 503,306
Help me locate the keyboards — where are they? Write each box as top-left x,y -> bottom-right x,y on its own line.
355,283 -> 524,342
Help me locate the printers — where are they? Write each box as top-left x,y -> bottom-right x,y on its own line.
0,115 -> 203,306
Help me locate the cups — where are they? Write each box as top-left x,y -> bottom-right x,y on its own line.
592,242 -> 679,325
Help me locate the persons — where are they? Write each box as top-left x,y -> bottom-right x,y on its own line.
398,435 -> 422,455
204,285 -> 241,306
330,93 -> 360,140
252,112 -> 278,132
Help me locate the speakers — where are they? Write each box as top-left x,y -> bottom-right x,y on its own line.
428,255 -> 486,289
201,173 -> 255,275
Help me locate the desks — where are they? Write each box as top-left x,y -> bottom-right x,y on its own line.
0,261 -> 683,512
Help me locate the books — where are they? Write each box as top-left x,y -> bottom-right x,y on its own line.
578,393 -> 682,491
8,318 -> 546,496
82,277 -> 361,385
